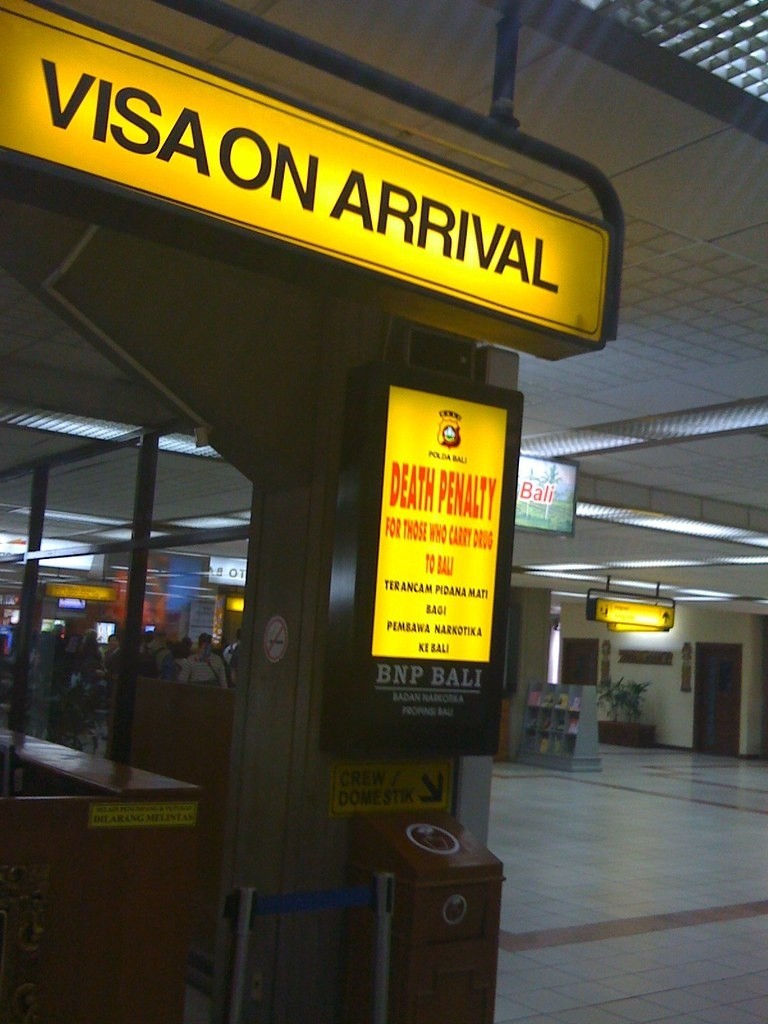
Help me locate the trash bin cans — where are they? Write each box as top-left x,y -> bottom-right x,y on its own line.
336,810 -> 506,1024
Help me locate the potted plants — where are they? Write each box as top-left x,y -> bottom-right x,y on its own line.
598,678 -> 655,747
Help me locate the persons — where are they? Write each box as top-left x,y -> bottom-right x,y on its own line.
29,624 -> 242,747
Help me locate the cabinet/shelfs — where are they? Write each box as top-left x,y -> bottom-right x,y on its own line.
516,682 -> 604,772
